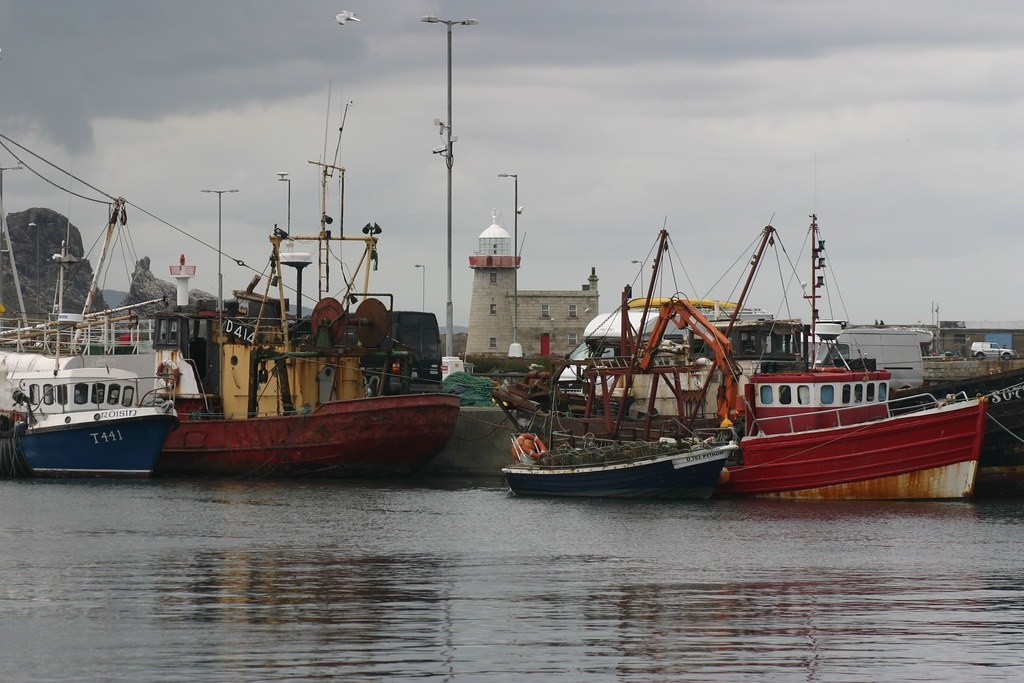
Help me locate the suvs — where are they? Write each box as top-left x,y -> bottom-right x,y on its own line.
970,341 -> 1015,361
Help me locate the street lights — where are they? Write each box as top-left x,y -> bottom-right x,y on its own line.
201,188 -> 240,398
414,264 -> 426,352
28,222 -> 41,320
497,172 -> 523,358
631,260 -> 644,298
420,14 -> 480,384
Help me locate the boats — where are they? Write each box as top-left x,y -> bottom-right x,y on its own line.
555,212 -> 936,399
0,126 -> 180,484
137,81 -> 461,478
715,211 -> 986,502
498,212 -> 744,504
888,364 -> 1024,477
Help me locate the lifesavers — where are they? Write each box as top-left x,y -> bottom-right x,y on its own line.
519,433 -> 546,458
156,360 -> 180,388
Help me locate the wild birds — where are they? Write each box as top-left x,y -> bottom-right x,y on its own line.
334,10 -> 361,25
